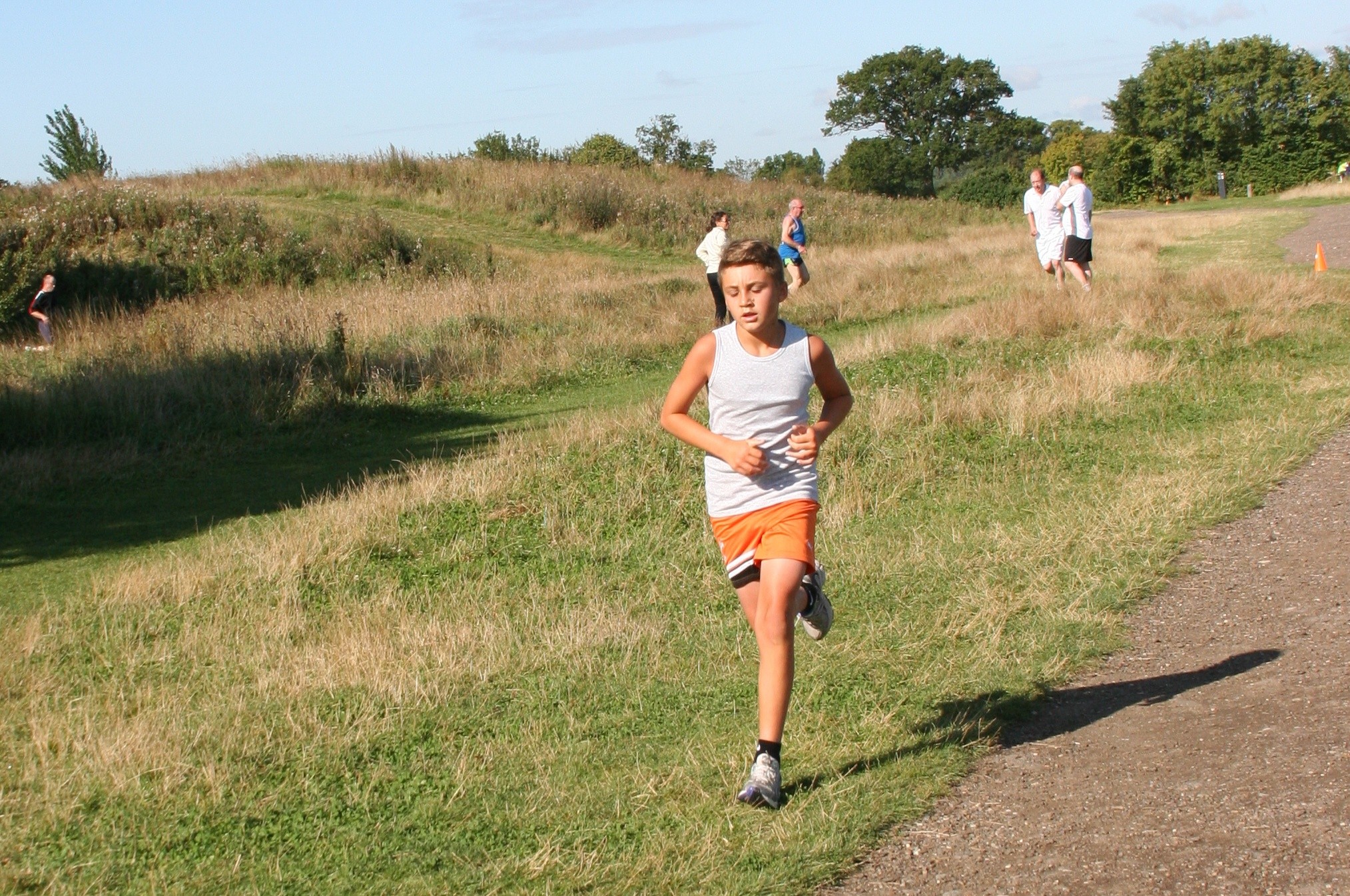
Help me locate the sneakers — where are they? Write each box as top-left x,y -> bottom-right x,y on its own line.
736,750 -> 781,808
796,559 -> 834,641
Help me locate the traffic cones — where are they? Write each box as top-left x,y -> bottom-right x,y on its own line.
1314,241 -> 1328,273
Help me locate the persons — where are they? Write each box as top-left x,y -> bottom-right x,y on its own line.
1023,168 -> 1065,289
655,239 -> 855,811
695,211 -> 734,329
1055,166 -> 1093,291
777,199 -> 809,290
